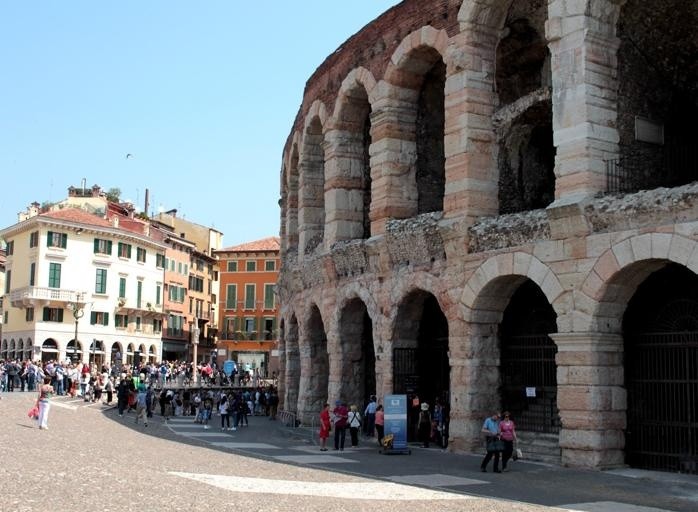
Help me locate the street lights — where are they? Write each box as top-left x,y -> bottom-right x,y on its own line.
66,291 -> 84,363
191,316 -> 201,382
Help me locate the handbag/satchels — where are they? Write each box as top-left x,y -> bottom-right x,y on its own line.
512,448 -> 522,461
486,436 -> 505,452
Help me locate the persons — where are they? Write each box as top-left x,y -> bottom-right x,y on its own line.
334,399 -> 347,451
374,403 -> 385,446
496,412 -> 518,471
0,353 -> 279,433
478,411 -> 502,473
319,403 -> 332,451
432,397 -> 443,446
416,401 -> 431,448
346,404 -> 361,448
364,394 -> 376,438
